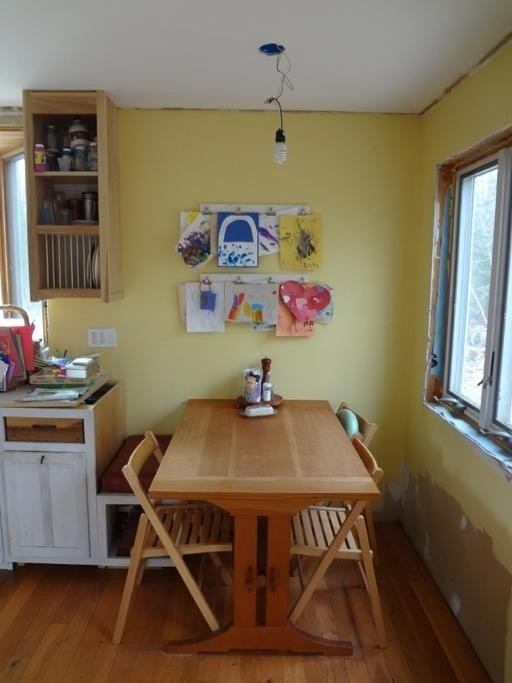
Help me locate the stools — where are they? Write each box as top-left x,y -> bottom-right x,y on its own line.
97,436 -> 197,569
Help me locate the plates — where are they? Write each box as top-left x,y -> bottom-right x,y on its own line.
85,244 -> 104,292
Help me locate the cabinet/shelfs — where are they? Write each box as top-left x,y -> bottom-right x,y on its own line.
22,90 -> 119,304
1,375 -> 122,572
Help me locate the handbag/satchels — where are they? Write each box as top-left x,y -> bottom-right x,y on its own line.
0,304 -> 40,392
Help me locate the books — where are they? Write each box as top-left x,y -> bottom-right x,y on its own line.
1,327 -> 104,404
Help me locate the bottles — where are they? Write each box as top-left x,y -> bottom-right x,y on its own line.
46,148 -> 59,171
75,145 -> 86,171
62,148 -> 72,172
44,125 -> 57,149
34,142 -> 47,172
37,190 -> 80,226
260,356 -> 274,402
68,119 -> 88,150
87,142 -> 98,172
81,190 -> 98,222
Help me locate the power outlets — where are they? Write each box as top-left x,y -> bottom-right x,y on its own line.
88,329 -> 118,347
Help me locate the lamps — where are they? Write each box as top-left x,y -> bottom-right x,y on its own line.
262,51 -> 297,167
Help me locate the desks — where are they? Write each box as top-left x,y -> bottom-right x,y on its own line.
148,399 -> 382,656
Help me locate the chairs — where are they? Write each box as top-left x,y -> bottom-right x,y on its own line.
110,429 -> 235,648
287,438 -> 388,655
334,399 -> 379,451
